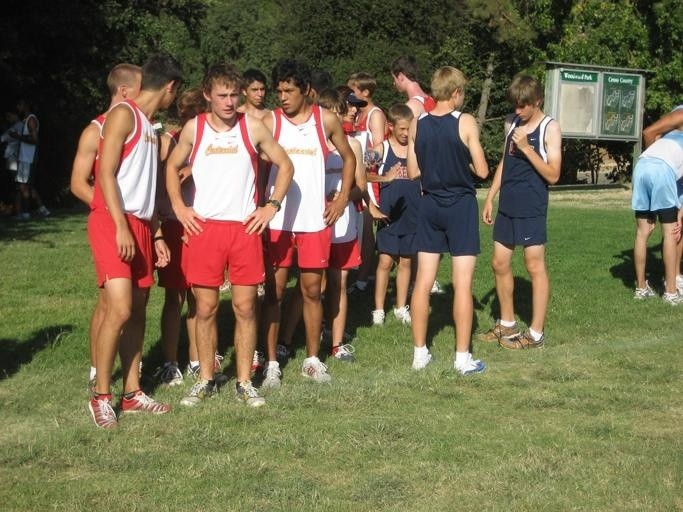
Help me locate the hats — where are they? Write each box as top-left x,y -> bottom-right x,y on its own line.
344,90 -> 369,109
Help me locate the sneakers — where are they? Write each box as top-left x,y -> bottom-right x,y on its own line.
331,345 -> 357,364
475,322 -> 521,342
393,304 -> 411,325
498,328 -> 547,350
300,355 -> 331,384
88,397 -> 119,431
661,274 -> 683,305
370,309 -> 387,325
234,381 -> 267,409
180,379 -> 220,408
453,359 -> 486,377
119,390 -> 171,415
265,360 -> 283,391
11,206 -> 51,221
633,281 -> 656,301
412,353 -> 431,370
432,280 -> 441,292
88,345 -> 292,384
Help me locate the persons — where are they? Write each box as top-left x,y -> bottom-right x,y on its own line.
7,97 -> 51,221
480,69 -> 562,352
628,125 -> 682,305
404,64 -> 491,376
69,59 -> 143,396
151,52 -> 445,412
641,102 -> 683,296
1,106 -> 24,217
84,46 -> 186,432
